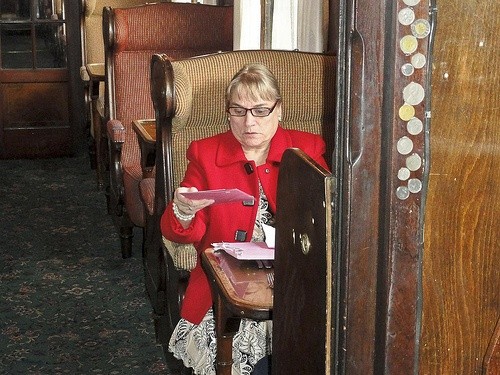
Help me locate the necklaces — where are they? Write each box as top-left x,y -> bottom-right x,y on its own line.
259,198 -> 264,230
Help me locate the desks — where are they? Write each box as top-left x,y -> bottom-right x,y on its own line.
85,63 -> 107,186
131,116 -> 158,169
200,247 -> 277,375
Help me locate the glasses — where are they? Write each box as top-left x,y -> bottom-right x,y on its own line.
226,99 -> 279,117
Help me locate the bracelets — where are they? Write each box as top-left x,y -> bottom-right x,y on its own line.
171,202 -> 195,222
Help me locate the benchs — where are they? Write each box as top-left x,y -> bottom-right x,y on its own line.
71,0 -> 232,259
140,48 -> 327,314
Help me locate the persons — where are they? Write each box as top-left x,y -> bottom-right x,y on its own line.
161,62 -> 331,375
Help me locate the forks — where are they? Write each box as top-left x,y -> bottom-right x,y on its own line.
267,273 -> 274,289
